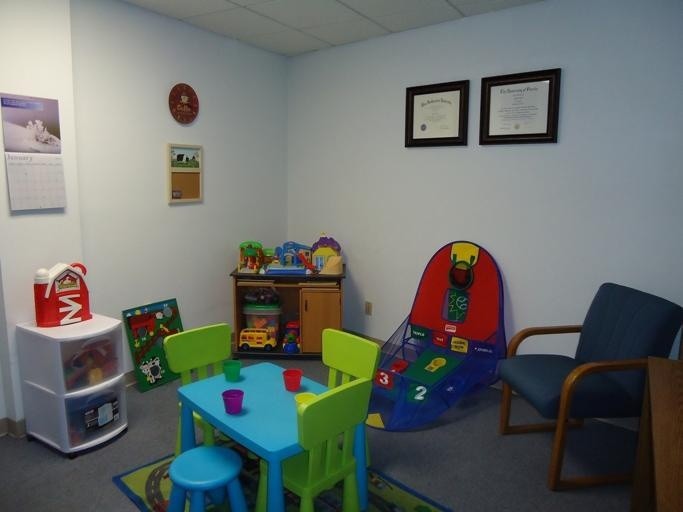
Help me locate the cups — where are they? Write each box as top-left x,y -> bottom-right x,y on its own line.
282,368 -> 303,392
222,390 -> 244,414
223,358 -> 242,381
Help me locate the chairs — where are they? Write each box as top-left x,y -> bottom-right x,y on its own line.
318,326 -> 380,471
251,378 -> 371,512
162,321 -> 233,456
496,280 -> 681,493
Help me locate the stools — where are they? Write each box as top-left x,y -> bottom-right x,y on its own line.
163,443 -> 249,511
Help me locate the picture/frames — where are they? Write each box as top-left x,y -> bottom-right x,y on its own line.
478,66 -> 562,146
403,80 -> 470,147
164,142 -> 204,206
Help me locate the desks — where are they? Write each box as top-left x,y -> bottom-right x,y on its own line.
633,353 -> 682,511
174,357 -> 372,511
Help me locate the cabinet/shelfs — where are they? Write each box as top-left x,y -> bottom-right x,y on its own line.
14,311 -> 128,460
229,264 -> 343,359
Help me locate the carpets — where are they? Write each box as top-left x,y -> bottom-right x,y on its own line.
111,432 -> 448,512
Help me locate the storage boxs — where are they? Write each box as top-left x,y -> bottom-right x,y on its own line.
242,302 -> 282,339
